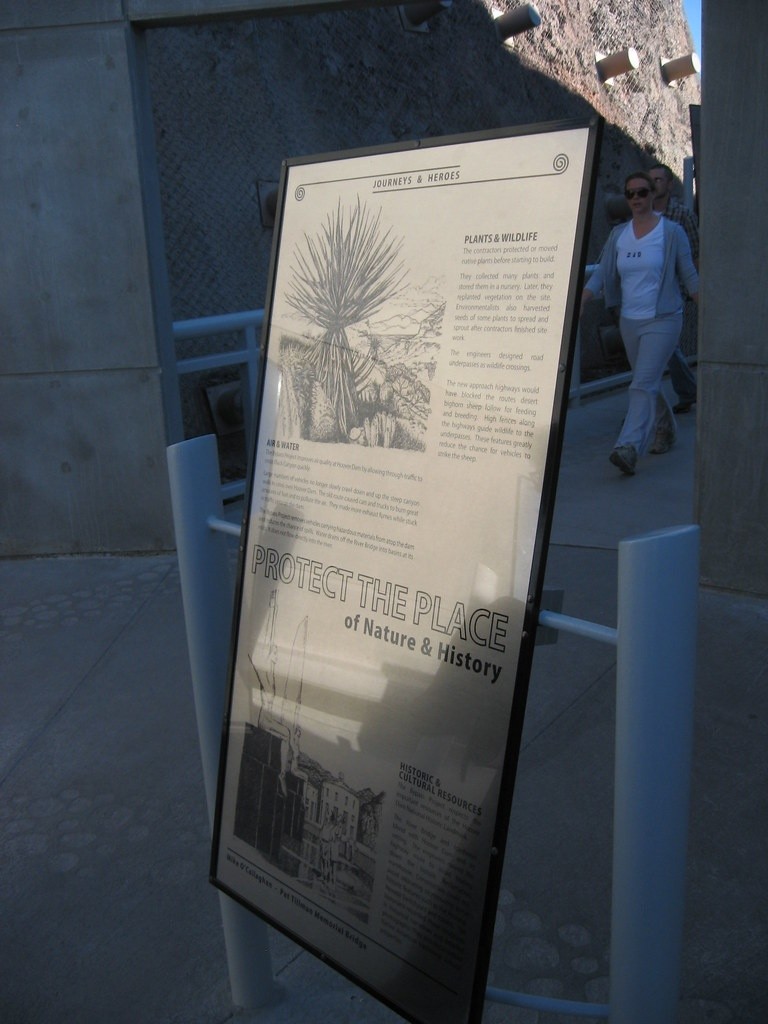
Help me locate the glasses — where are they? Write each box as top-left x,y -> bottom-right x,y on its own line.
624,187 -> 652,199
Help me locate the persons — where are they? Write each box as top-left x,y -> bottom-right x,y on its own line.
579,163 -> 699,474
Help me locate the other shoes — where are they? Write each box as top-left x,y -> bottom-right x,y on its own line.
610,446 -> 637,476
649,431 -> 678,453
672,391 -> 697,413
